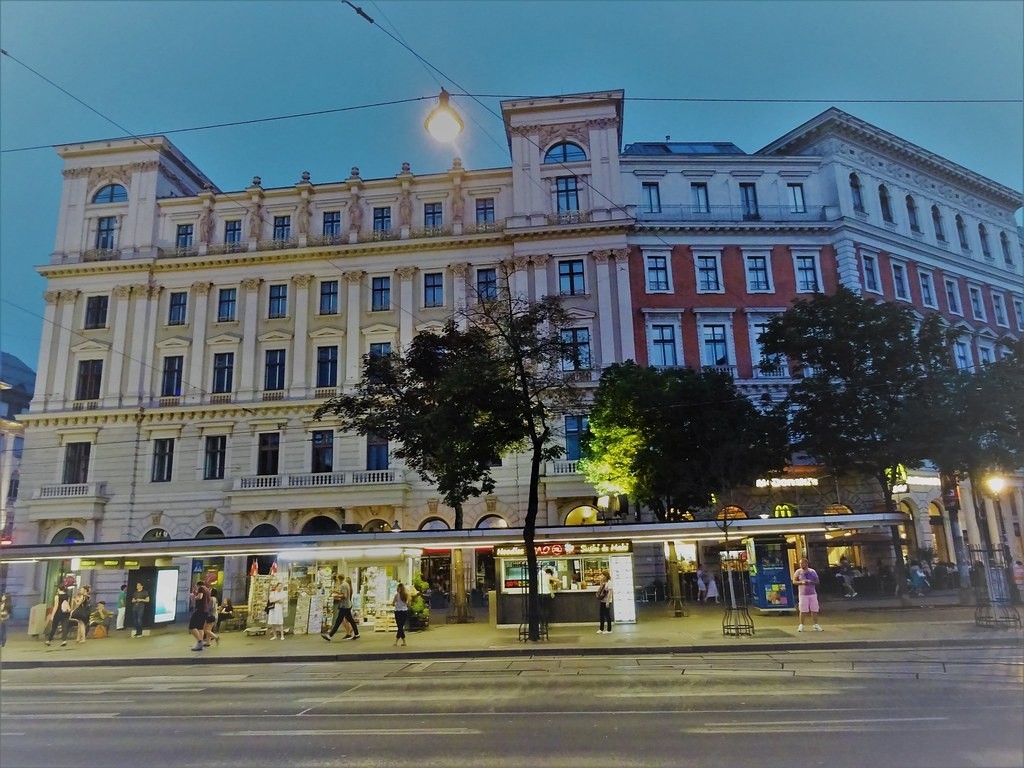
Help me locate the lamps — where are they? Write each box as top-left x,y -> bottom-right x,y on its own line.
425,91 -> 464,141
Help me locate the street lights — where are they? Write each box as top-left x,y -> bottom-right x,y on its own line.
989,479 -> 1023,608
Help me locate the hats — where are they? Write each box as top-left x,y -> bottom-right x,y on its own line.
601,571 -> 611,580
57,585 -> 65,590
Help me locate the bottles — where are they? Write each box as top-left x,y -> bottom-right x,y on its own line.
582,581 -> 586,589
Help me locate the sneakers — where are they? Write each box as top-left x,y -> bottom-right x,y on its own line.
797,624 -> 805,632
812,624 -> 824,632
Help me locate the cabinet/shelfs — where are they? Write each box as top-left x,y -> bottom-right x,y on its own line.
375,601 -> 407,631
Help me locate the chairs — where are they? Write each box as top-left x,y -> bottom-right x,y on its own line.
634,583 -> 669,603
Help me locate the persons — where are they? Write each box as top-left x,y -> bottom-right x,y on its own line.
248,205 -> 263,240
838,556 -> 982,598
0,593 -> 12,647
452,189 -> 465,221
793,558 -> 824,632
198,209 -> 215,243
348,194 -> 364,230
595,571 -> 614,634
696,564 -> 721,604
189,581 -> 233,652
398,191 -> 414,225
321,574 -> 360,641
294,200 -> 313,232
131,582 -> 150,638
392,583 -> 410,646
45,583 -> 111,647
537,561 -> 561,629
115,585 -> 128,631
268,582 -> 285,641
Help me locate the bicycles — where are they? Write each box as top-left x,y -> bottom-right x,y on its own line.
893,569 -> 933,596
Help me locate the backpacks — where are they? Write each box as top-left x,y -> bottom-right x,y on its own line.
60,598 -> 71,614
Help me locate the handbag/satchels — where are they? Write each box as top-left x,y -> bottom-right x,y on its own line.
86,625 -> 108,639
405,602 -> 414,617
69,602 -> 89,624
267,602 -> 275,609
206,613 -> 216,623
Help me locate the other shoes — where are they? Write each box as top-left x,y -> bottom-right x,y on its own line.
76,638 -> 85,643
596,629 -> 613,634
844,594 -> 851,597
350,634 -> 359,640
270,637 -> 276,640
281,637 -> 284,641
851,592 -> 858,598
60,641 -> 67,645
45,640 -> 51,645
342,634 -> 352,640
321,634 -> 332,641
194,636 -> 221,652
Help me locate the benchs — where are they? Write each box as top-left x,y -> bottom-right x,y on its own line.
212,605 -> 248,631
66,612 -> 114,639
